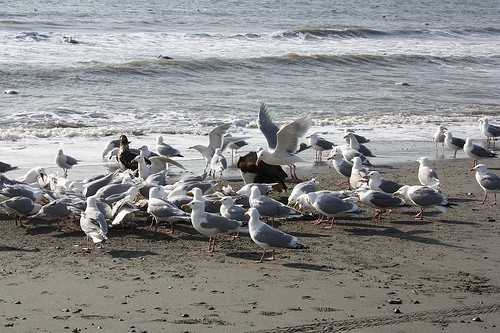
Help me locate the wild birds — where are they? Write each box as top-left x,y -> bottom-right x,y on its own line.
0,102 -> 500,264
